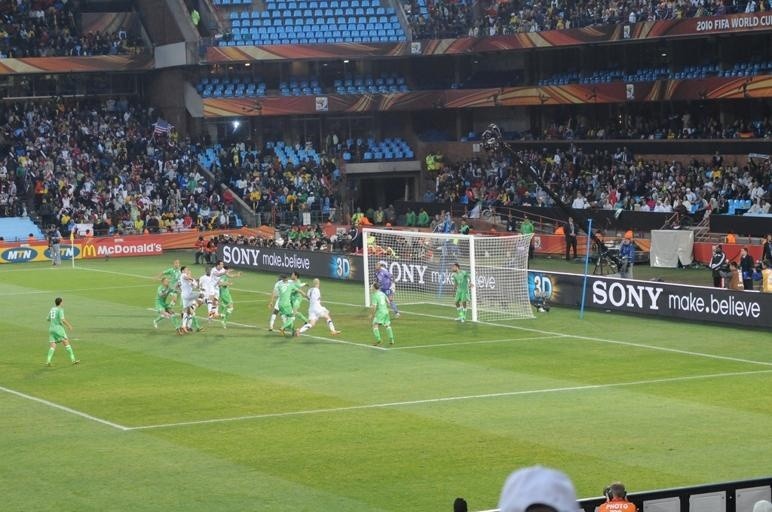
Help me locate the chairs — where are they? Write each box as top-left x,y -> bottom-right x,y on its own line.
212,0 -> 407,46
333,72 -> 410,94
537,57 -> 772,86
267,141 -> 320,167
720,199 -> 752,216
195,78 -> 267,97
279,75 -> 323,96
198,144 -> 257,170
342,136 -> 415,162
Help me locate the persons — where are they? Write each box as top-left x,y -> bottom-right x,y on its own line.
153,257 -> 341,338
368,282 -> 394,347
373,256 -> 401,321
45,297 -> 80,368
1,0 -> 772,291
454,496 -> 469,512
598,481 -> 637,512
451,262 -> 472,323
